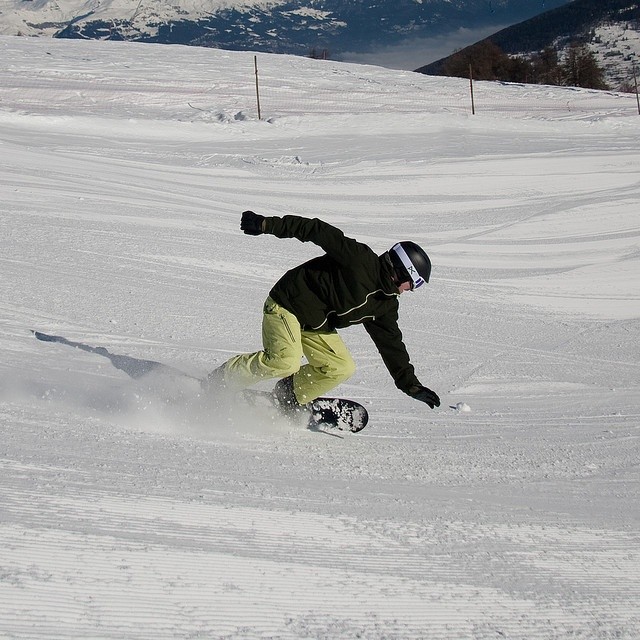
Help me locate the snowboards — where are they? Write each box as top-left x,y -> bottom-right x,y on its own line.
235,389 -> 368,433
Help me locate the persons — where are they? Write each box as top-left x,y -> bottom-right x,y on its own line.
202,210 -> 441,430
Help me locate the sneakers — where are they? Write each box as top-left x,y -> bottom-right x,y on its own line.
275,375 -> 308,430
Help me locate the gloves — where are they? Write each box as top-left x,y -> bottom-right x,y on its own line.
240,211 -> 267,236
403,380 -> 441,409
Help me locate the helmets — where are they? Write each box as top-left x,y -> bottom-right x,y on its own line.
389,240 -> 432,284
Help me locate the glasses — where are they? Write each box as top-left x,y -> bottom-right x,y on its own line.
393,242 -> 427,292
